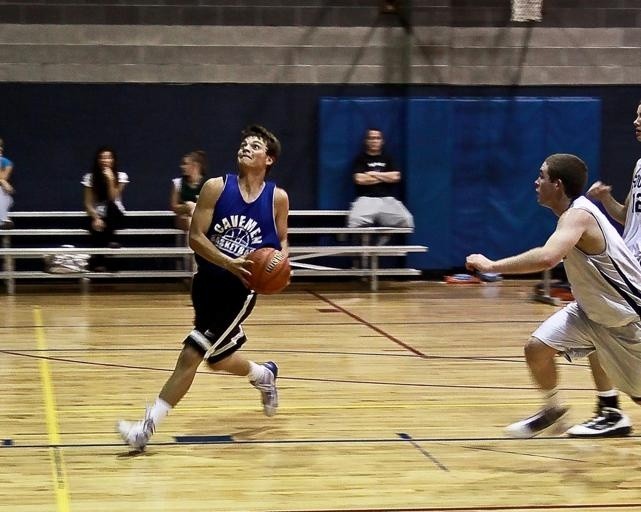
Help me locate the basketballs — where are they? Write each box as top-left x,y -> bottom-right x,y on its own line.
245,248 -> 290,295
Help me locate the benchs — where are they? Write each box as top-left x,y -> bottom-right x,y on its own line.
0,209 -> 430,291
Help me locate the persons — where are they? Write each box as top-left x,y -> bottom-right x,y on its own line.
462,153 -> 641,437
116,125 -> 293,453
0,140 -> 19,225
562,102 -> 641,439
81,151 -> 131,249
348,127 -> 416,246
171,151 -> 209,253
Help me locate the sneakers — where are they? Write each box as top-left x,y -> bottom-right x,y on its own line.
95,266 -> 106,272
108,241 -> 120,247
254,359 -> 278,418
509,405 -> 567,439
569,406 -> 633,436
118,418 -> 155,450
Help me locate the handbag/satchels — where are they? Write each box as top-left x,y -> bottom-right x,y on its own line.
44,245 -> 91,275
